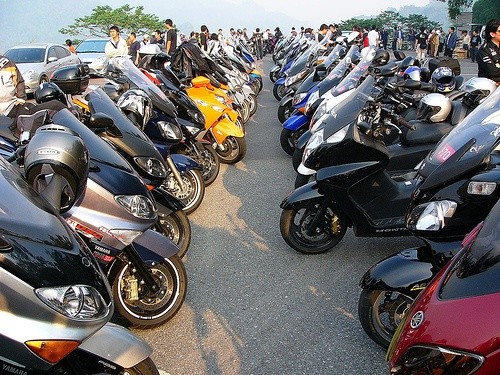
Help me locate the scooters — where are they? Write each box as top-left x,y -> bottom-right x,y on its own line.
0,34 -> 264,375
279,68 -> 500,256
357,83 -> 500,350
269,33 -> 498,190
385,197 -> 500,375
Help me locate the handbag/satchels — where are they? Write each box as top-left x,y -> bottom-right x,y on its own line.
420,43 -> 427,49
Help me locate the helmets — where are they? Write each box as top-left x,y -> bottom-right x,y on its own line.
24,124 -> 90,213
420,93 -> 451,122
117,89 -> 153,130
150,53 -> 170,70
374,50 -> 390,65
404,66 -> 421,81
431,67 -> 456,92
466,77 -> 497,105
35,82 -> 68,107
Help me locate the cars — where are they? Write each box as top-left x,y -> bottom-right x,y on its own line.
75,37 -> 112,79
3,42 -> 82,94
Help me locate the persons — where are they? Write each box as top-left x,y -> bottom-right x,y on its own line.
408,26 -> 445,62
274,27 -> 282,41
444,27 -> 457,58
291,28 -> 297,36
164,19 -> 177,56
252,28 -> 265,60
181,35 -> 187,42
140,34 -> 150,48
66,40 -> 77,55
105,26 -> 128,57
469,29 -> 481,63
263,29 -> 270,39
476,19 -> 500,87
191,25 -> 250,58
460,29 -> 470,50
130,32 -> 140,67
300,24 -> 406,51
151,30 -> 163,44
0,55 -> 27,117
